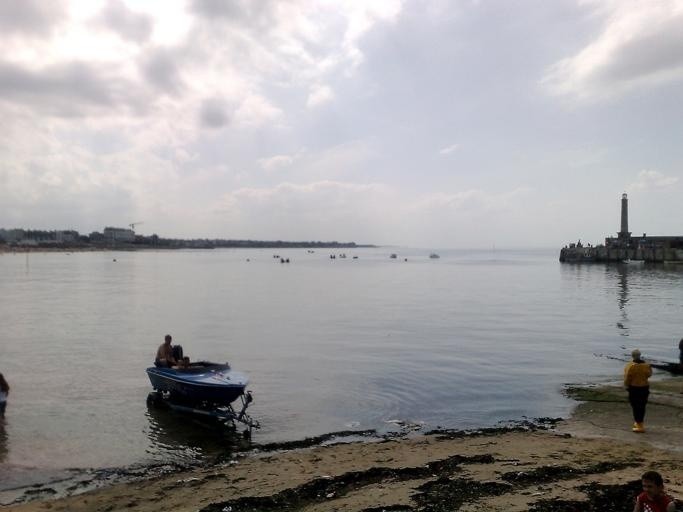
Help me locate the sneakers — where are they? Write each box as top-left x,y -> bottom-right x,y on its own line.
632,422 -> 645,432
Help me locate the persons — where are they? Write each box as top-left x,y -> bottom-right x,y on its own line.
171,356 -> 205,369
624,349 -> 652,433
632,472 -> 676,512
154,335 -> 173,365
0,372 -> 10,418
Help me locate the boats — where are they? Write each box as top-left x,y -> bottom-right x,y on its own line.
146,362 -> 261,431
